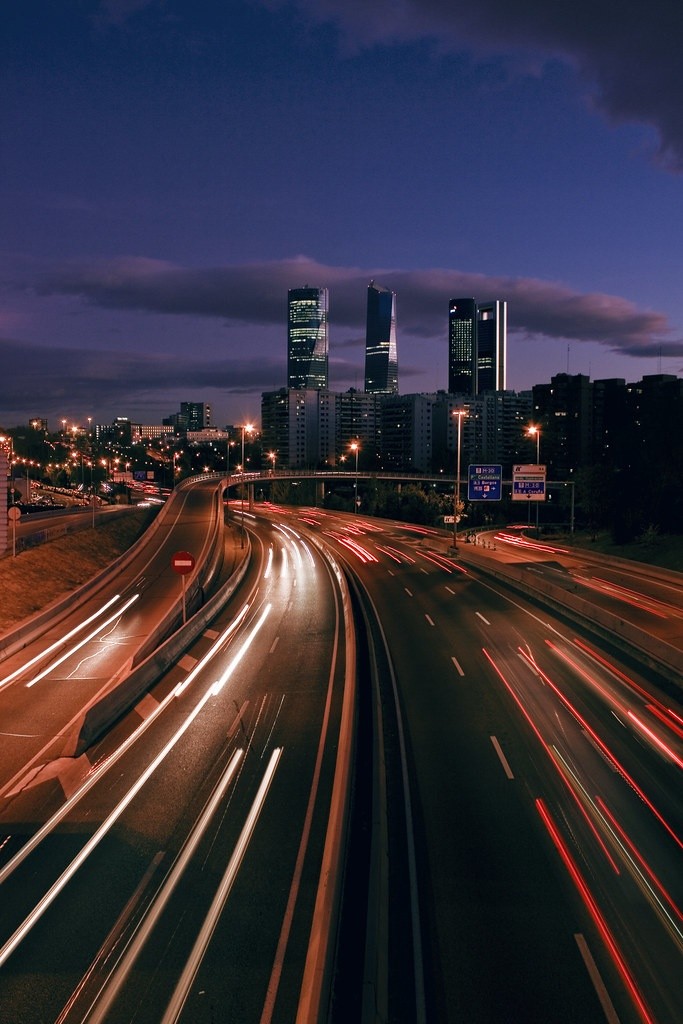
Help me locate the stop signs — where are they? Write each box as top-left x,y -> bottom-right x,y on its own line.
171,550 -> 196,575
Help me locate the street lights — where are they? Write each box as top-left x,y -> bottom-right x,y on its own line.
173,454 -> 180,488
88,462 -> 93,483
351,443 -> 359,515
270,452 -> 277,505
101,459 -> 107,500
109,458 -> 120,504
240,424 -> 253,550
125,462 -> 131,505
226,441 -> 235,526
529,426 -> 540,539
452,408 -> 468,548
